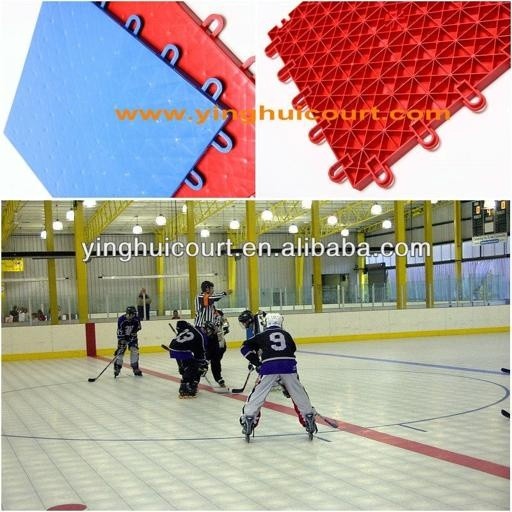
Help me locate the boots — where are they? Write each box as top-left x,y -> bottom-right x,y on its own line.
114,369 -> 120,376
133,368 -> 143,375
190,386 -> 197,396
179,385 -> 190,395
241,417 -> 254,435
306,417 -> 317,433
217,377 -> 225,385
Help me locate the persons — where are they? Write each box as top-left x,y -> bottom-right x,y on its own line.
112,306 -> 143,376
168,281 -> 233,397
9,304 -> 47,322
239,313 -> 317,434
239,309 -> 298,399
136,288 -> 152,320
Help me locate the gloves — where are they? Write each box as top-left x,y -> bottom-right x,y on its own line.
198,359 -> 209,377
248,362 -> 255,371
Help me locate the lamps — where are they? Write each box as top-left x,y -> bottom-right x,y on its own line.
40,200 -> 393,242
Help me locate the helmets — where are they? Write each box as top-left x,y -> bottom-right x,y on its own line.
200,280 -> 215,288
264,312 -> 285,329
126,306 -> 137,314
202,320 -> 215,331
238,310 -> 255,322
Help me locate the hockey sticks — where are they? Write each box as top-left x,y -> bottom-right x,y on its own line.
169,323 -> 229,393
501,409 -> 509,417
232,368 -> 252,392
501,367 -> 509,374
88,340 -> 130,382
276,379 -> 338,427
